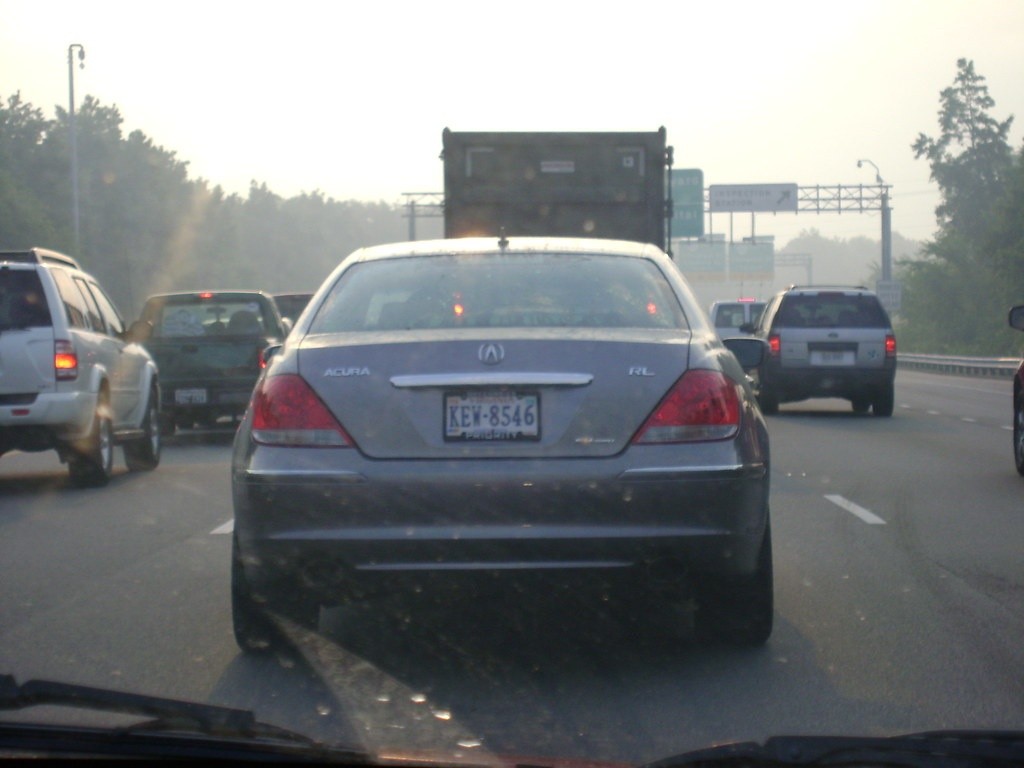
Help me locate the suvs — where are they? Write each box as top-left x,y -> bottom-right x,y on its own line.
138,289 -> 289,439
1,248 -> 166,483
740,284 -> 900,416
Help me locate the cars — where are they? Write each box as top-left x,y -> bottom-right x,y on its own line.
227,233 -> 781,647
257,294 -> 312,326
709,301 -> 774,366
1006,302 -> 1024,473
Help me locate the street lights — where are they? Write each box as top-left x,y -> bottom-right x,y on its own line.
67,43 -> 86,259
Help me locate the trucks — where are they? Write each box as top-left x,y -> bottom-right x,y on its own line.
440,120 -> 676,264
